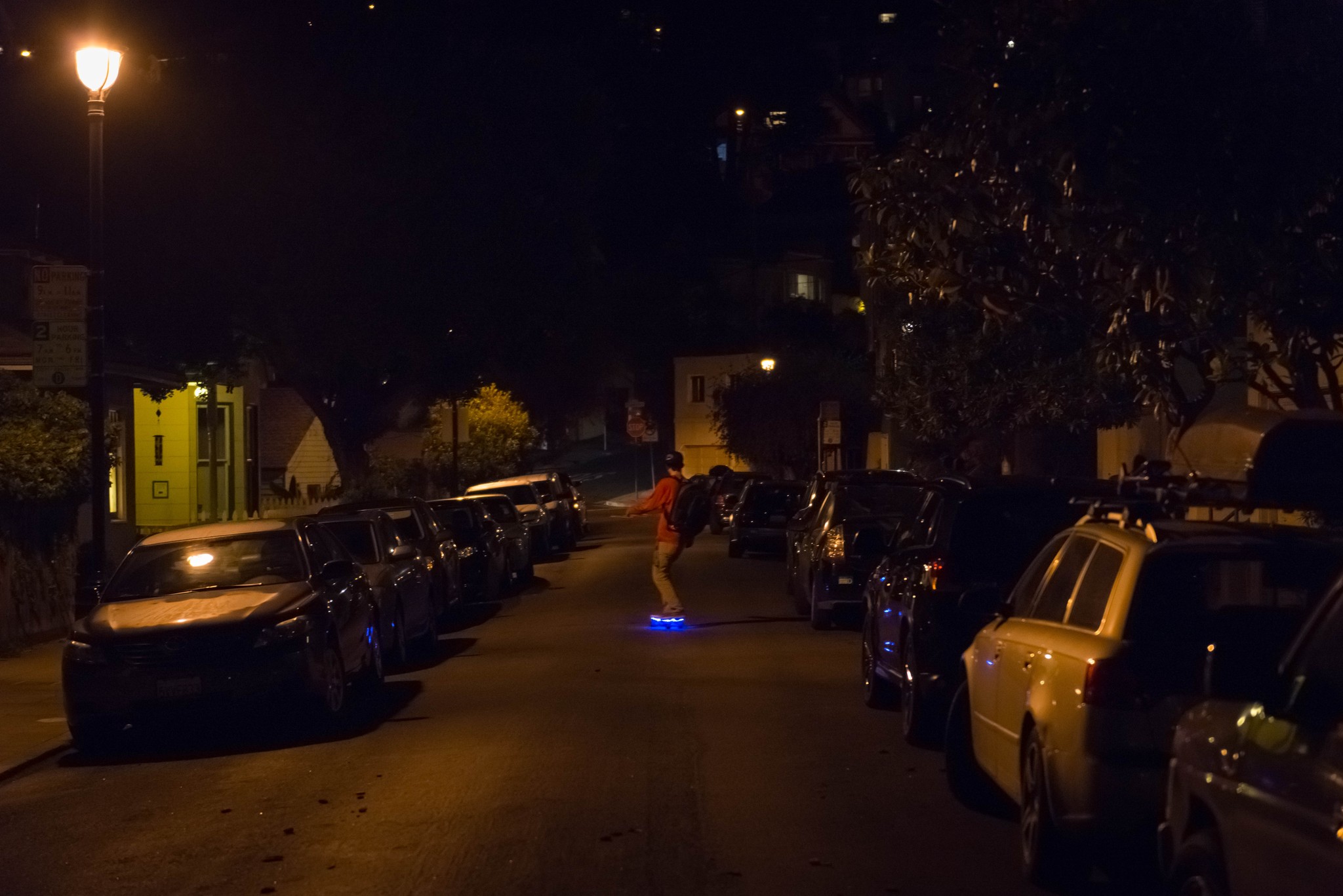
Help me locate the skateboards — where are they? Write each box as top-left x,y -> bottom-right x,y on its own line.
649,612 -> 685,630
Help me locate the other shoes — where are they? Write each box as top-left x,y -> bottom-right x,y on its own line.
662,605 -> 686,614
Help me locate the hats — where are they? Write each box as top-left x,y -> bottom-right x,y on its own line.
664,451 -> 683,465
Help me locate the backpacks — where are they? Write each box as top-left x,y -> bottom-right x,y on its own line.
661,473 -> 712,536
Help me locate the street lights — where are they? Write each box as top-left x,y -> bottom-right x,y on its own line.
74,2 -> 129,626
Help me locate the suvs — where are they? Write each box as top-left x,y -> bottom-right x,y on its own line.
851,474 -> 1164,745
949,405 -> 1343,893
496,472 -> 574,549
463,478 -> 552,554
704,462 -> 971,629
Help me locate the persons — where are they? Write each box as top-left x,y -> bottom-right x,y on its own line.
625,452 -> 693,619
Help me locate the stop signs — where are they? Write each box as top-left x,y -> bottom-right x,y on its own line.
627,417 -> 646,437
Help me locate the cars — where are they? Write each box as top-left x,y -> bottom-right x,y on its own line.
563,481 -> 590,536
424,497 -> 508,602
61,517 -> 384,746
1162,574 -> 1343,896
558,473 -> 583,487
289,508 -> 437,665
462,494 -> 536,581
316,496 -> 463,623
